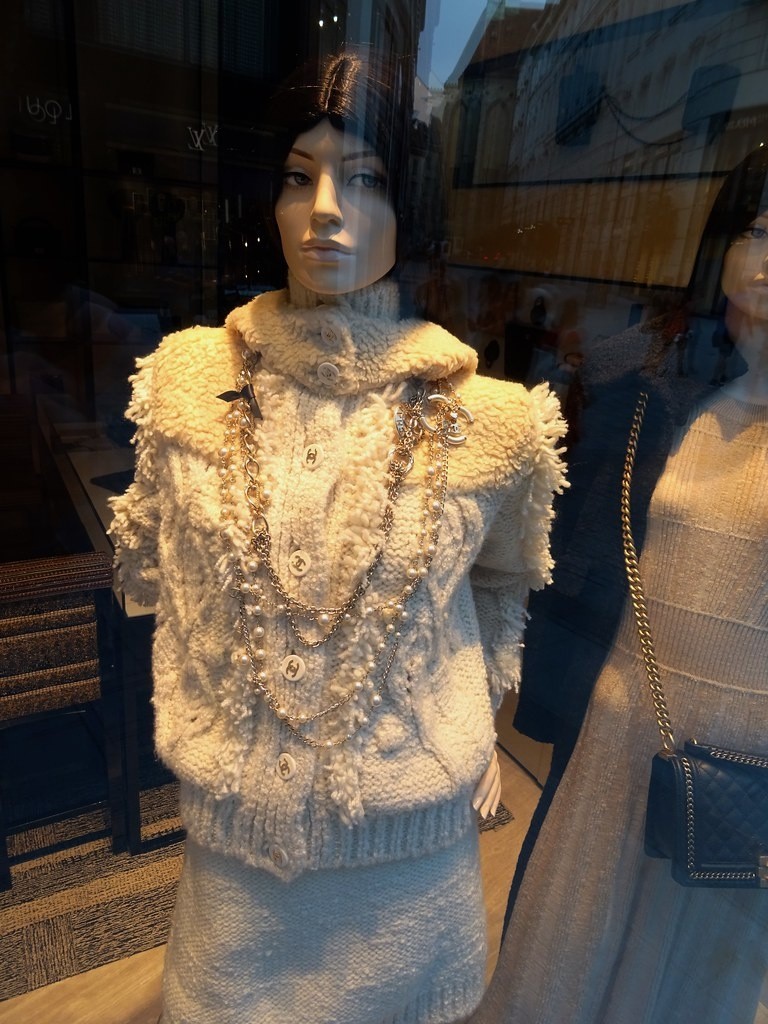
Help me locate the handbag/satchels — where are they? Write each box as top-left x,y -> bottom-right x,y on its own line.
645,739 -> 768,891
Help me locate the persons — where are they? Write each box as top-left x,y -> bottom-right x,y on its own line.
108,53 -> 572,1024
468,150 -> 767,1023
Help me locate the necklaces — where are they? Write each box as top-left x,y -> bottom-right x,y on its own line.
230,337 -> 473,748
